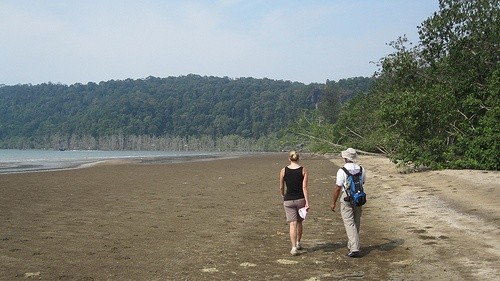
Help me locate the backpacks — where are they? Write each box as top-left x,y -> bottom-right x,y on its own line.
340,165 -> 366,207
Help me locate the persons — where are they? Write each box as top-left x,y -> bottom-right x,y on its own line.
280,151 -> 310,255
331,148 -> 366,257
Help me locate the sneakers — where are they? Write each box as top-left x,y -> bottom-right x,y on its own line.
346,251 -> 359,258
347,241 -> 351,249
290,250 -> 298,256
296,245 -> 301,250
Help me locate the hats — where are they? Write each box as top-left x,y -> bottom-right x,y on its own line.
341,148 -> 359,162
